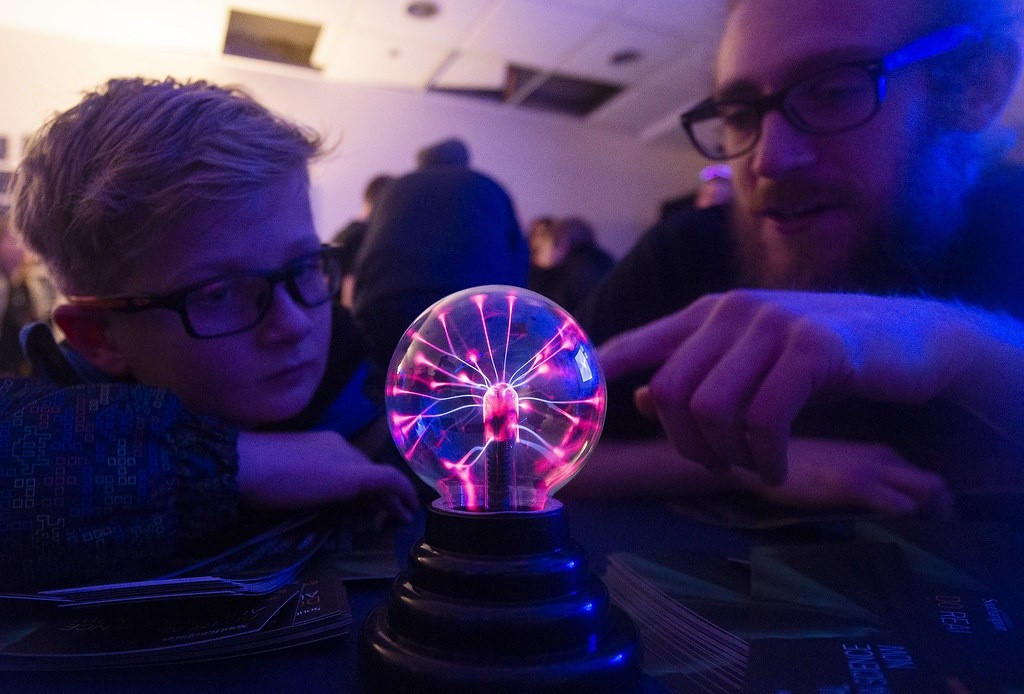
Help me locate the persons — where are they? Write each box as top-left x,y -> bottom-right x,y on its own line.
658,163 -> 734,215
0,76 -> 415,505
324,136 -> 532,415
375,0 -> 1024,513
528,216 -> 617,317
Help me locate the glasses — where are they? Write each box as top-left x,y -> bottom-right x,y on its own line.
680,16 -> 994,163
68,243 -> 353,340
